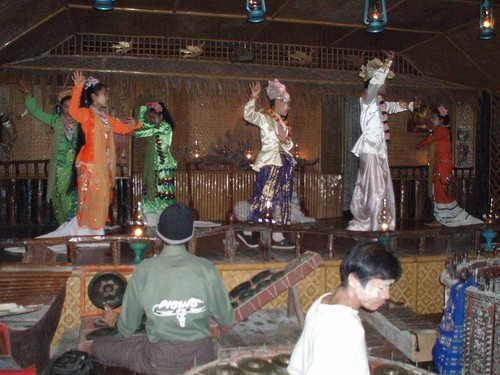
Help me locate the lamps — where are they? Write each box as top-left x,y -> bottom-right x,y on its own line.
479,0 -> 497,39
363,0 -> 387,32
244,141 -> 253,171
128,210 -> 147,263
191,140 -> 203,171
245,0 -> 267,22
482,198 -> 499,252
93,0 -> 114,9
377,200 -> 393,246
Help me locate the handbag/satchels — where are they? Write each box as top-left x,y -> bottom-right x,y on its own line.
432,274 -> 473,375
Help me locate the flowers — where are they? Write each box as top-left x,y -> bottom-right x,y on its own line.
265,78 -> 290,103
378,95 -> 391,142
437,105 -> 448,116
84,76 -> 99,90
266,108 -> 293,141
57,89 -> 72,102
145,100 -> 163,113
356,56 -> 395,81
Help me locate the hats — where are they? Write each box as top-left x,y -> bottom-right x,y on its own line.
156,203 -> 194,245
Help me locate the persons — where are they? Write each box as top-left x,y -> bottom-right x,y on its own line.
69,69 -> 144,235
125,101 -> 176,224
346,48 -> 421,231
284,239 -> 402,375
416,103 -> 484,227
91,204 -> 235,374
235,77 -> 299,250
17,83 -> 79,227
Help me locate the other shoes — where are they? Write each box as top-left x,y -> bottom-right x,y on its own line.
271,238 -> 296,249
237,231 -> 259,247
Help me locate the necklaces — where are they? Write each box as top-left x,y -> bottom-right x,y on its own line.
268,108 -> 292,141
378,95 -> 391,141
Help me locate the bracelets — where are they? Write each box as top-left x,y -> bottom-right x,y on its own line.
251,95 -> 259,98
